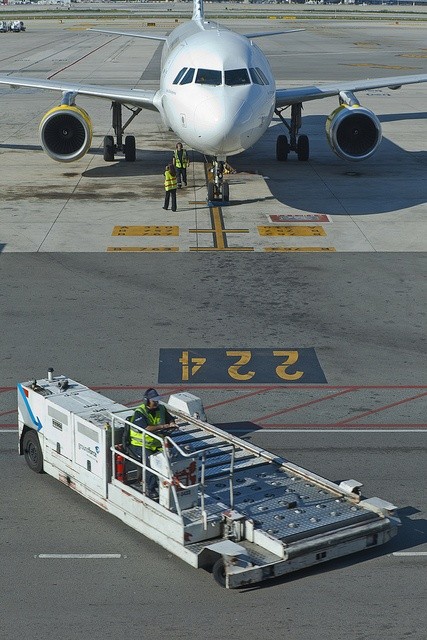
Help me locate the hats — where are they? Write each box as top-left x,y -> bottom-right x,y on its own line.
145,389 -> 163,401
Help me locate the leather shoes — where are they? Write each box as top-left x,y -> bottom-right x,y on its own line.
150,490 -> 159,500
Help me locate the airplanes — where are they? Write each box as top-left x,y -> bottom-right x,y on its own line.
0,0 -> 427,203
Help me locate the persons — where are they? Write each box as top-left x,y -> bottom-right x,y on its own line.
172,142 -> 190,189
163,162 -> 178,212
129,387 -> 180,501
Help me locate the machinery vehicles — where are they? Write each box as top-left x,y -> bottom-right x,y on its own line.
16,368 -> 402,590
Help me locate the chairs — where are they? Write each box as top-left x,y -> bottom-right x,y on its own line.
121,416 -> 142,491
